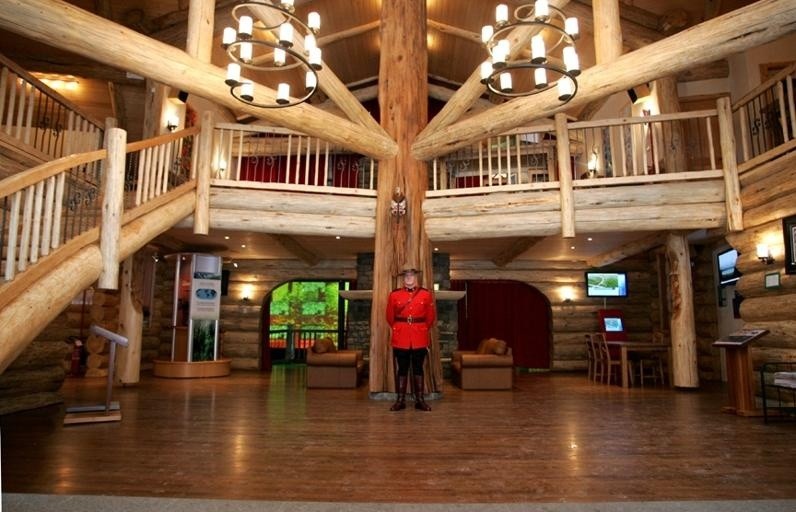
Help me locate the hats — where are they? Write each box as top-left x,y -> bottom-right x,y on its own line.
394,264 -> 422,275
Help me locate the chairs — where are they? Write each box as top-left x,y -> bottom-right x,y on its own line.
761,359 -> 796,426
582,330 -> 671,387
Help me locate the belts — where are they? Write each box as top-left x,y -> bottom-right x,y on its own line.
393,316 -> 424,323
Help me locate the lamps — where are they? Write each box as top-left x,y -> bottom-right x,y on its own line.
221,0 -> 324,110
479,0 -> 583,112
757,243 -> 773,262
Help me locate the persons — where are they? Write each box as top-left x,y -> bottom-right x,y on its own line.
385,264 -> 436,411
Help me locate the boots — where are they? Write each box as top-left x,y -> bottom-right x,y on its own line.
390,377 -> 407,412
413,376 -> 430,411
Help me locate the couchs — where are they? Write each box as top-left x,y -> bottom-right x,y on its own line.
305,334 -> 366,390
450,335 -> 515,390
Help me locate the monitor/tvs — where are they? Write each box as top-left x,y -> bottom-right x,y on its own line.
717,248 -> 742,285
586,272 -> 628,297
603,316 -> 624,332
